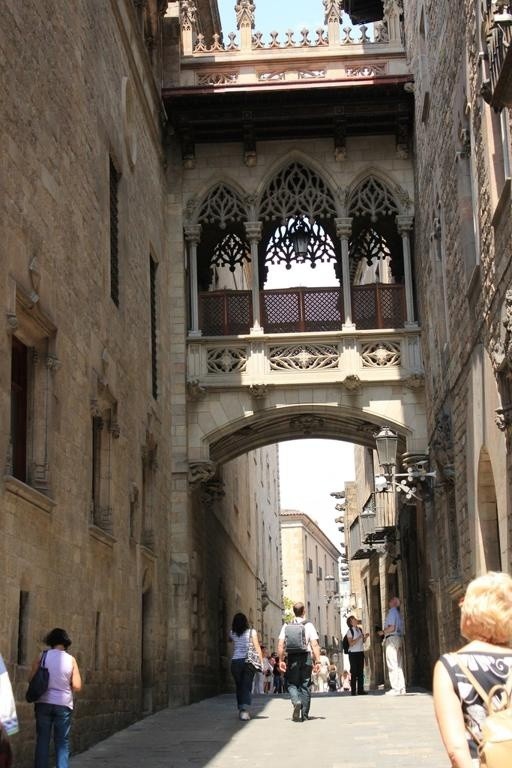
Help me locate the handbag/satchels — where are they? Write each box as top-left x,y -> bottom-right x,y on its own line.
24,648 -> 49,703
244,628 -> 263,673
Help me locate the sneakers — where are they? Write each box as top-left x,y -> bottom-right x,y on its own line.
351,689 -> 368,695
293,700 -> 309,722
384,687 -> 406,695
240,711 -> 250,721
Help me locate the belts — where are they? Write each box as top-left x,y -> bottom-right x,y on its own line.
385,634 -> 401,639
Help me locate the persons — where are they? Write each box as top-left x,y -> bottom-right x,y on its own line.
277,601 -> 322,721
227,611 -> 266,721
345,614 -> 371,695
1,647 -> 21,767
251,646 -> 354,693
432,570 -> 512,768
376,595 -> 409,695
27,626 -> 82,767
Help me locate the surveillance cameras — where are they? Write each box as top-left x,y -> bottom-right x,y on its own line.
415,460 -> 428,474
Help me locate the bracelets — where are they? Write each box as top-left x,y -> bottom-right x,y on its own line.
383,629 -> 386,633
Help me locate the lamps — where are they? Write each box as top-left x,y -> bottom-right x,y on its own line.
371,428 -> 436,500
361,507 -> 400,546
325,574 -> 352,604
289,216 -> 309,262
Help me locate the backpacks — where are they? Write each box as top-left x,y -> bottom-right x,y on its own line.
450,650 -> 511,768
342,628 -> 354,654
284,619 -> 310,654
